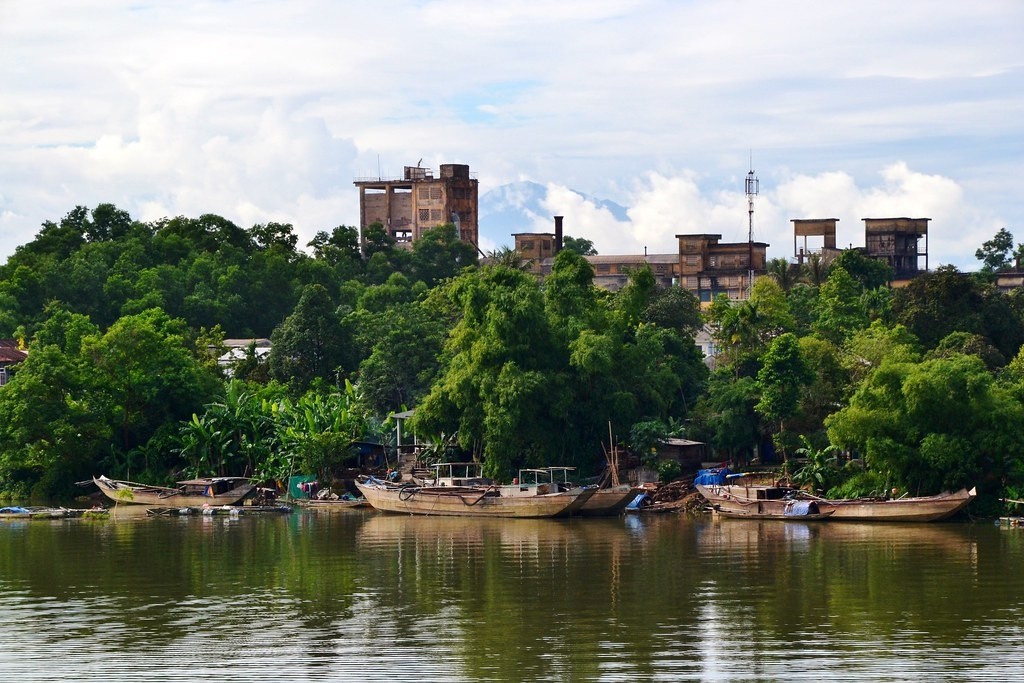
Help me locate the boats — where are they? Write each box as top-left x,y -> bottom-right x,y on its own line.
29,506 -> 107,519
712,501 -> 835,520
304,499 -> 370,508
354,421 -> 679,520
694,468 -> 977,523
92,475 -> 263,506
146,507 -> 181,518
